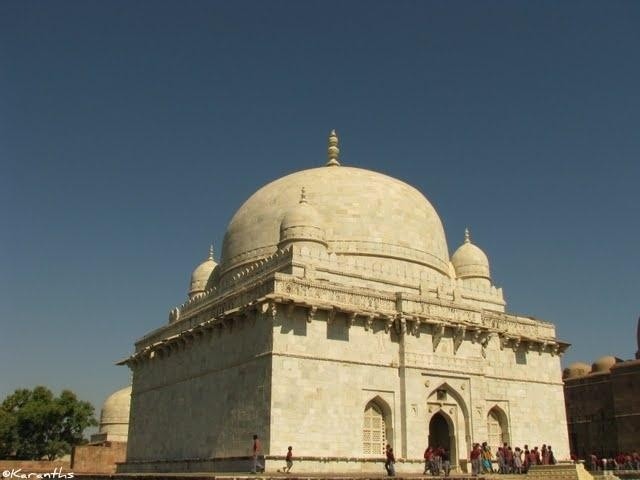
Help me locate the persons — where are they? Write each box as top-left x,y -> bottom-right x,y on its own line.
283,446 -> 294,472
249,435 -> 265,475
571,450 -> 640,470
470,442 -> 555,474
424,443 -> 451,476
384,444 -> 396,476
